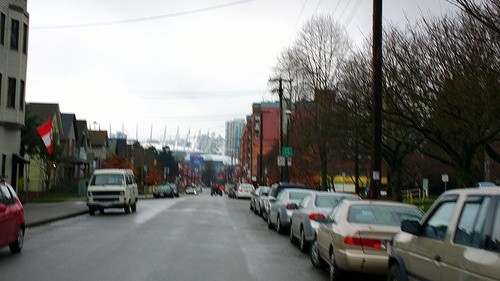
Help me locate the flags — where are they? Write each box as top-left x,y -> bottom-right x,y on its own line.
36,112 -> 65,155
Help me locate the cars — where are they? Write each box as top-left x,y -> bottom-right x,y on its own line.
185,187 -> 202,195
310,198 -> 437,281
224,183 -> 255,199
153,183 -> 179,198
250,185 -> 270,217
287,192 -> 364,254
267,188 -> 318,234
0,180 -> 26,253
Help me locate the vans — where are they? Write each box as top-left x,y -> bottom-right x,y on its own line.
85,169 -> 138,216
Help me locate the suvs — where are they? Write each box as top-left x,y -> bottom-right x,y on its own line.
387,185 -> 500,281
262,182 -> 307,221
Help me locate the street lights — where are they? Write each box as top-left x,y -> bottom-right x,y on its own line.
283,110 -> 292,182
